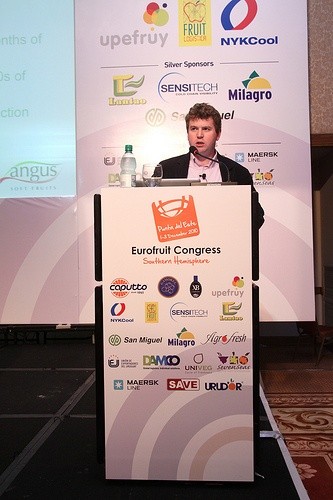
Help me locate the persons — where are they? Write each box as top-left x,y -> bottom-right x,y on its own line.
152,103 -> 266,229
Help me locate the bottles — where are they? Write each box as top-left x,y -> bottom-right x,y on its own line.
120,145 -> 136,187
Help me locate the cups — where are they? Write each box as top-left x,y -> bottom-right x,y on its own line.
142,164 -> 163,187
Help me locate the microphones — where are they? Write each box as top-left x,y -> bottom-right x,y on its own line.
189,146 -> 230,183
